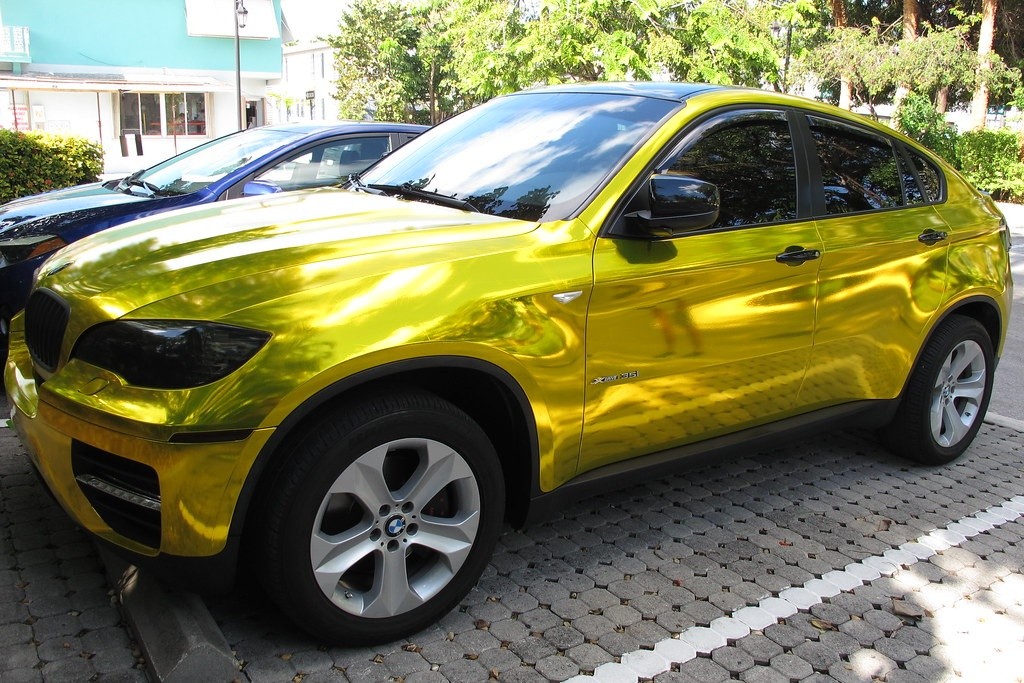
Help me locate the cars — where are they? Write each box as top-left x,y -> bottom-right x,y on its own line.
5,82 -> 1014,649
0,122 -> 432,341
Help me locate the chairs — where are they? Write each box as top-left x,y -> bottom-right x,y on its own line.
340,149 -> 358,177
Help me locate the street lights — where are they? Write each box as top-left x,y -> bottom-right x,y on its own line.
233,0 -> 249,130
770,11 -> 793,92
